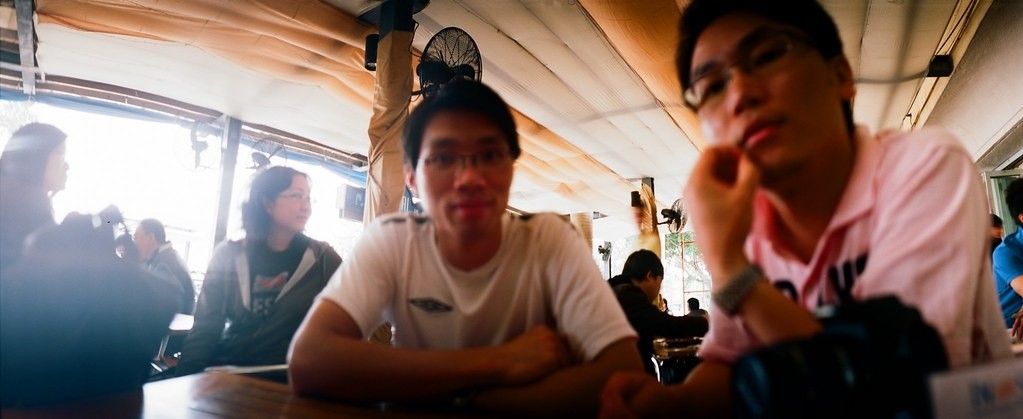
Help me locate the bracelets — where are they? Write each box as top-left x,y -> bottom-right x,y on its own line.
712,266 -> 761,317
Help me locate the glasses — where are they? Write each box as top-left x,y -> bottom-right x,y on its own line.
276,193 -> 316,205
685,39 -> 804,111
418,150 -> 510,176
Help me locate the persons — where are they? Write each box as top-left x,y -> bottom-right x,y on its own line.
286,79 -> 645,419
0,122 -> 195,408
600,2 -> 1016,418
173,165 -> 342,383
985,177 -> 1022,343
600,247 -> 709,387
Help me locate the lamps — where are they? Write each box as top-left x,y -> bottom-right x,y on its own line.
365,33 -> 379,72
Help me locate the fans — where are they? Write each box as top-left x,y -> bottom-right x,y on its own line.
410,28 -> 482,107
183,120 -> 223,171
654,198 -> 688,235
598,239 -> 613,262
236,135 -> 290,175
